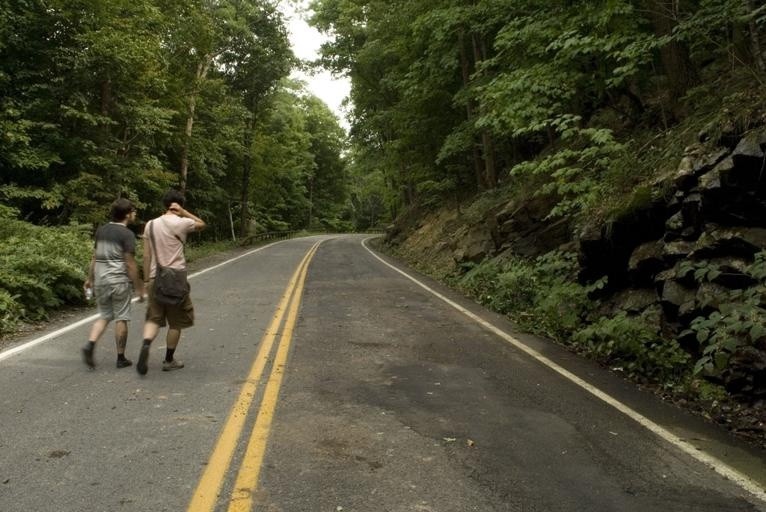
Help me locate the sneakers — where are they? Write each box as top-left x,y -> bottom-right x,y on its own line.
117,359 -> 132,368
137,345 -> 149,375
162,359 -> 184,371
82,344 -> 94,366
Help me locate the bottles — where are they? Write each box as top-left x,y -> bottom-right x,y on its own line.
85,282 -> 93,301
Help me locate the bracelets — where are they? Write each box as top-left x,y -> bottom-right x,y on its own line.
144,281 -> 149,282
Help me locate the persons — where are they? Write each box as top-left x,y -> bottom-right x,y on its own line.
81,197 -> 145,368
137,189 -> 206,375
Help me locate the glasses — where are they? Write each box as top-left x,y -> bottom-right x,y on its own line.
129,208 -> 138,213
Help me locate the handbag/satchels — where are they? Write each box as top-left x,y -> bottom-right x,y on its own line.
152,263 -> 190,308
